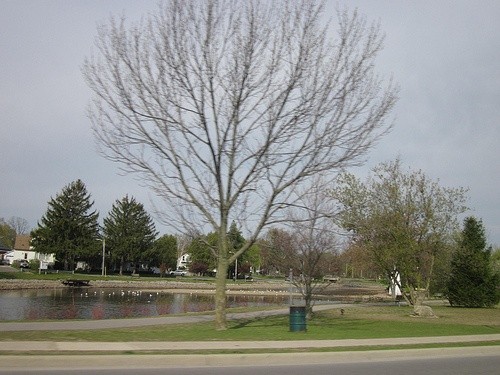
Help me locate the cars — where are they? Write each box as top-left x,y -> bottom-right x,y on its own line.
168,269 -> 187,277
18,259 -> 29,269
232,272 -> 251,280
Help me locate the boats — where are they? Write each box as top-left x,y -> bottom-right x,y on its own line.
62,279 -> 90,286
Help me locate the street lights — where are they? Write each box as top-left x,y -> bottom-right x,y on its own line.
228,251 -> 238,280
96,238 -> 105,275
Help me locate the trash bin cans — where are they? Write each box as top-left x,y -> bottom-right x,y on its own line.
289,304 -> 307,333
